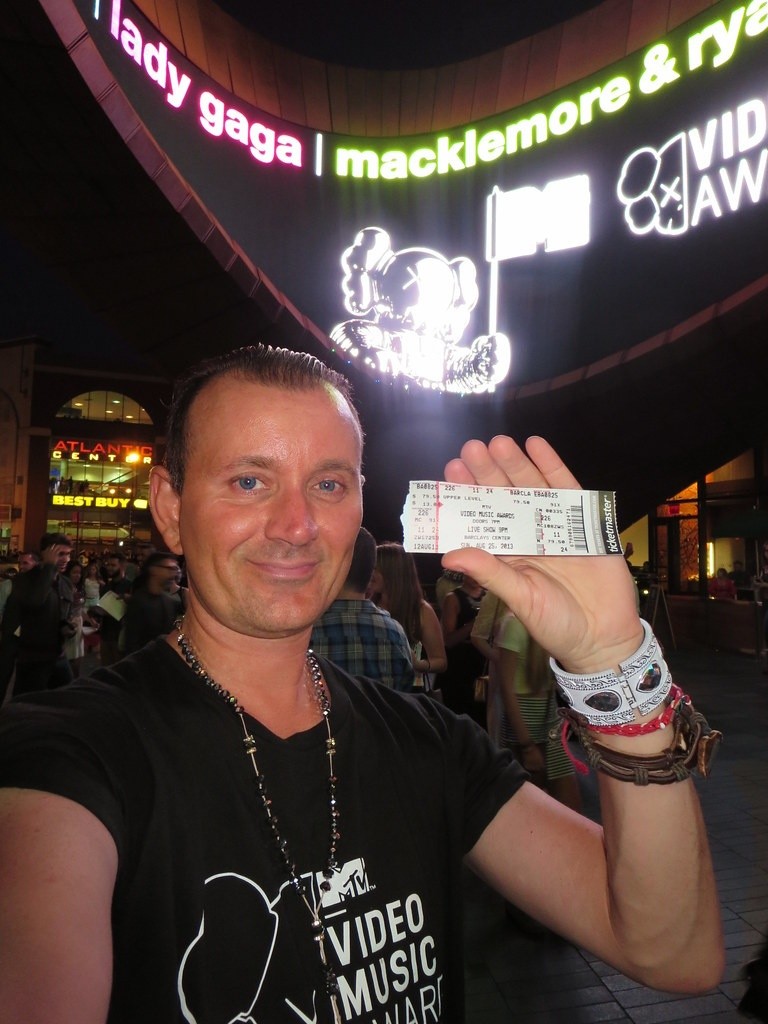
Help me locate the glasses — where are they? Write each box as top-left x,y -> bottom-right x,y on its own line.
154,563 -> 179,572
54,549 -> 72,559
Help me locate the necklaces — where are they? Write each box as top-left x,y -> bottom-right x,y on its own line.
174,614 -> 341,998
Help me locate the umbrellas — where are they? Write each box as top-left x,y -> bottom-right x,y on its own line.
711,508 -> 768,578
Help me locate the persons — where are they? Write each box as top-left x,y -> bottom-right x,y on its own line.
0,525 -> 655,813
1,343 -> 723,1024
709,560 -> 750,600
753,540 -> 768,674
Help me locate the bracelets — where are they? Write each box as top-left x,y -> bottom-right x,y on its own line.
546,619 -> 723,789
